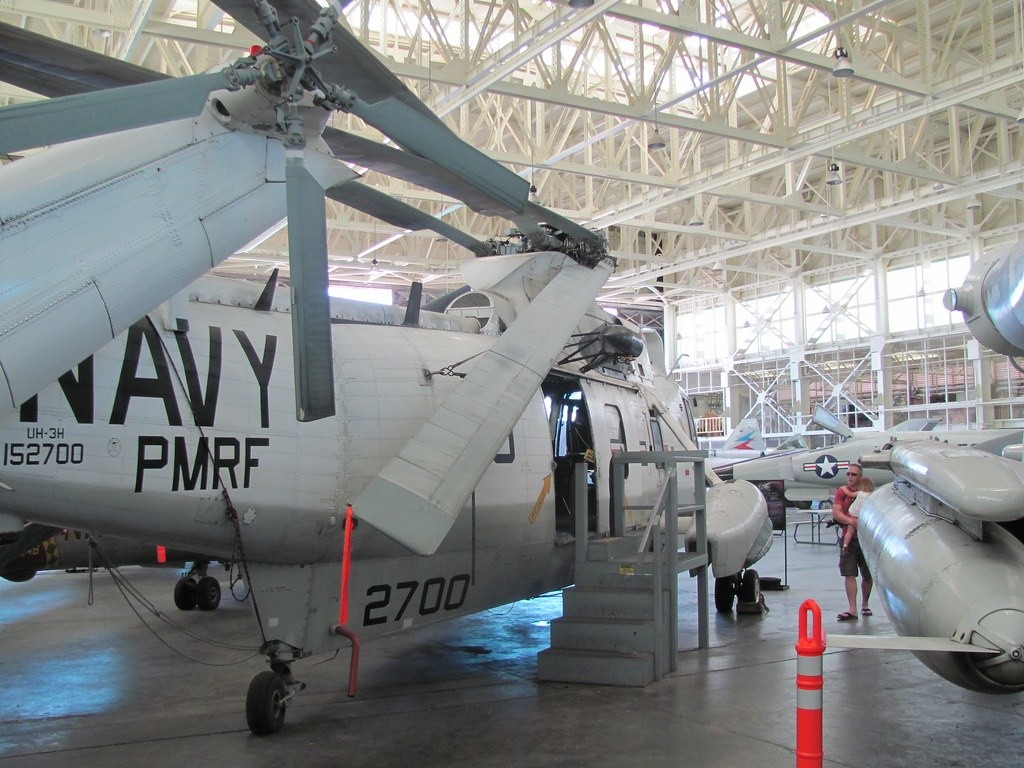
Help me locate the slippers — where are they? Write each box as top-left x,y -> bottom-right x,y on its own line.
861,608 -> 872,616
837,612 -> 858,620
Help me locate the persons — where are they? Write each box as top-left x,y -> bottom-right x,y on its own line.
832,463 -> 875,620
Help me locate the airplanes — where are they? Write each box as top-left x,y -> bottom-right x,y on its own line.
825,240 -> 1023,695
1,0 -> 774,735
708,419 -> 765,473
734,402 -> 1024,513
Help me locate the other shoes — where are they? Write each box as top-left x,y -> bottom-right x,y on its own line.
843,544 -> 848,558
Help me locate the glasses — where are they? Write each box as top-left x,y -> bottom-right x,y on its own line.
847,472 -> 861,476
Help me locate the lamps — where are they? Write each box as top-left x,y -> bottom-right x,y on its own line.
568,0 -> 595,8
711,205 -> 724,272
647,35 -> 666,149
528,142 -> 540,203
689,194 -> 702,226
823,74 -> 843,187
965,112 -> 982,212
370,222 -> 379,275
436,234 -> 448,243
832,1 -> 855,78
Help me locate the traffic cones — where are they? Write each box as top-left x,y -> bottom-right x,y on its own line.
795,599 -> 826,768
